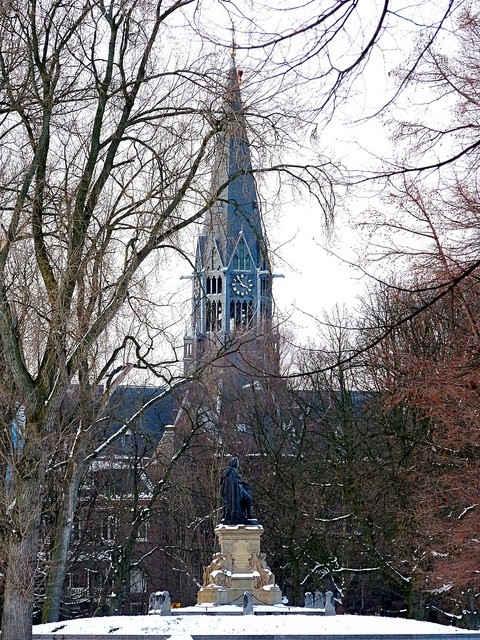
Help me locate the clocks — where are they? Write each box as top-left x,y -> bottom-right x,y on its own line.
231,273 -> 255,297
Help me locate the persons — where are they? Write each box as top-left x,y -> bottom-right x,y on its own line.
216,456 -> 261,526
